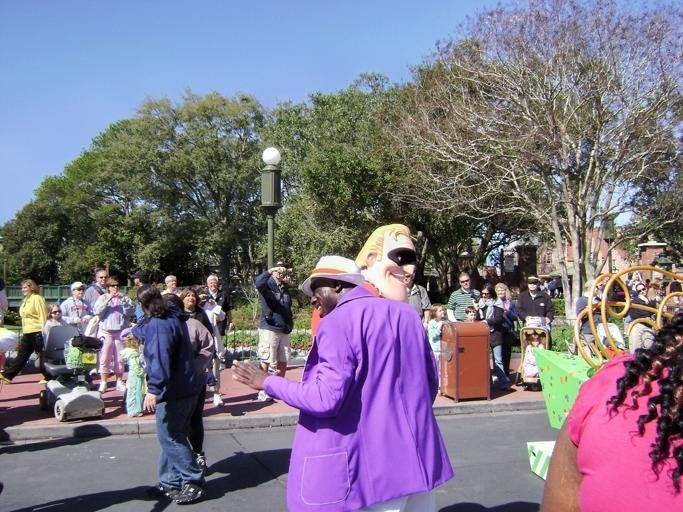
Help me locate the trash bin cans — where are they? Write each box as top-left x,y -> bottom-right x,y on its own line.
440,321 -> 490,402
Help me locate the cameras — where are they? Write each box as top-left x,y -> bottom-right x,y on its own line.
279,272 -> 284,279
204,294 -> 212,301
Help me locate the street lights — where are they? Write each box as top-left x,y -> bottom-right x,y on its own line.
259,146 -> 283,270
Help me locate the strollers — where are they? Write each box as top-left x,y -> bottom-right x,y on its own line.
515,325 -> 552,387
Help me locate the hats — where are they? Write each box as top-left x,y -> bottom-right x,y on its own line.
301,255 -> 367,297
271,260 -> 288,269
70,281 -> 86,291
527,274 -> 538,281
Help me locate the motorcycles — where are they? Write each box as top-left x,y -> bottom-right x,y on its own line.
39,323 -> 105,423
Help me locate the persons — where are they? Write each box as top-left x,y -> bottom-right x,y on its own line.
231,255 -> 454,512
311,223 -> 418,349
540,311 -> 683,512
405,268 -> 683,392
1,272 -> 227,504
255,261 -> 294,402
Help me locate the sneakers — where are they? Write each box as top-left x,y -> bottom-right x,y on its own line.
131,412 -> 144,417
0,372 -> 13,385
172,479 -> 209,505
257,389 -> 273,402
193,452 -> 211,476
98,379 -> 107,393
155,479 -> 183,500
213,393 -> 225,408
38,379 -> 49,384
504,382 -> 513,391
210,386 -> 219,392
116,380 -> 128,391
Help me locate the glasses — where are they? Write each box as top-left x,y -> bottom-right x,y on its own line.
405,275 -> 413,278
459,278 -> 470,283
50,310 -> 62,314
481,292 -> 490,295
74,287 -> 86,291
636,288 -> 646,293
107,283 -> 120,287
528,282 -> 537,284
97,276 -> 108,279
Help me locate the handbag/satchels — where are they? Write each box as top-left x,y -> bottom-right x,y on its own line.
264,308 -> 273,322
84,315 -> 100,338
